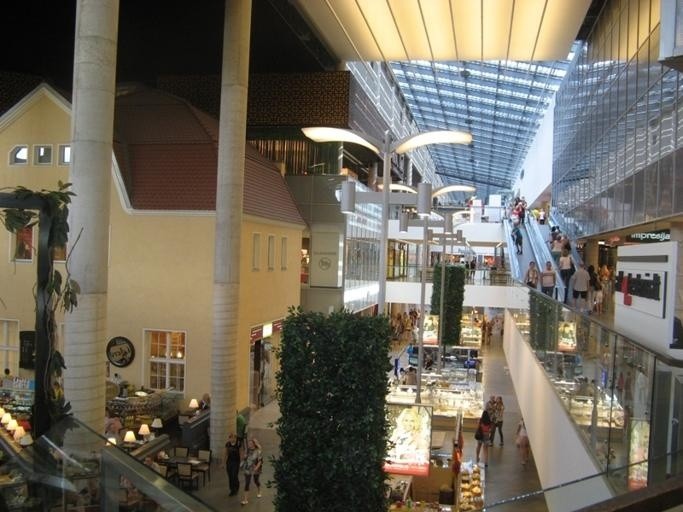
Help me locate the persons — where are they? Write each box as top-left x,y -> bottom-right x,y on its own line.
464,260 -> 477,277
482,319 -> 504,345
549,225 -> 572,283
0,367 -> 265,512
525,260 -> 556,297
510,195 -> 527,255
396,308 -> 418,328
516,420 -> 530,467
533,207 -> 546,225
569,263 -> 610,315
474,396 -> 505,468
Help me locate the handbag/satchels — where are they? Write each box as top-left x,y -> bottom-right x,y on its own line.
570,263 -> 576,274
475,426 -> 483,440
526,280 -> 532,286
565,242 -> 571,251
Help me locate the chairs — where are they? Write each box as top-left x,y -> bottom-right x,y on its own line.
119,445 -> 214,512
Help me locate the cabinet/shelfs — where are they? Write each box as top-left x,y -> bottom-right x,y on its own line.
384,315 -> 488,512
518,317 -> 631,440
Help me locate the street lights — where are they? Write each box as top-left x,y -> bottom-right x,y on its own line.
377,180 -> 475,403
300,125 -> 472,316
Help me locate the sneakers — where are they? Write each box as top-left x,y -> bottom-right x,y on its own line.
256,493 -> 262,498
240,499 -> 249,505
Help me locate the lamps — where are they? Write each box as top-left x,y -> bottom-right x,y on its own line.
105,419 -> 164,454
189,399 -> 199,416
0,408 -> 33,451
